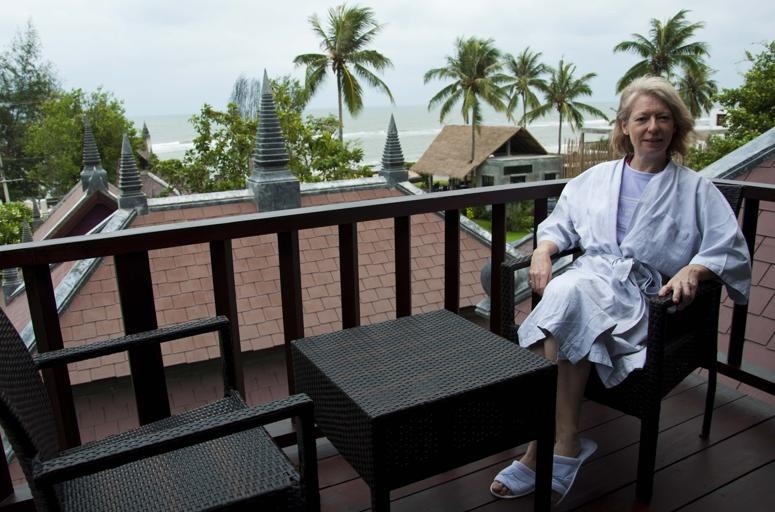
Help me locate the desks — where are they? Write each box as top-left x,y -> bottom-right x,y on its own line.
292,309 -> 559,512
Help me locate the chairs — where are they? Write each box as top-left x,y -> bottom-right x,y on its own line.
2,310 -> 322,511
498,182 -> 745,512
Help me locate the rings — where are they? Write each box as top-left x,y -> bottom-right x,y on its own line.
529,281 -> 535,288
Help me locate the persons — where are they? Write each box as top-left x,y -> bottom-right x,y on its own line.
491,76 -> 753,508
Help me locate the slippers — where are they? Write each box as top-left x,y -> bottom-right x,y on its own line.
551,434 -> 598,505
489,459 -> 536,499
